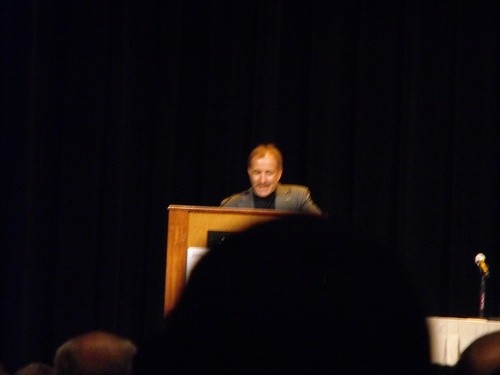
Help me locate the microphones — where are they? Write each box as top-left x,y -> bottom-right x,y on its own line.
474,252 -> 491,277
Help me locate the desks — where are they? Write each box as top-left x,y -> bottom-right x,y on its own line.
163,204 -> 291,320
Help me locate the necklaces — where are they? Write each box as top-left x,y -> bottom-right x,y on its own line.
266,193 -> 274,209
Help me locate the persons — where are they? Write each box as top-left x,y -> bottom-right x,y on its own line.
218,142 -> 322,214
16,195 -> 500,374
